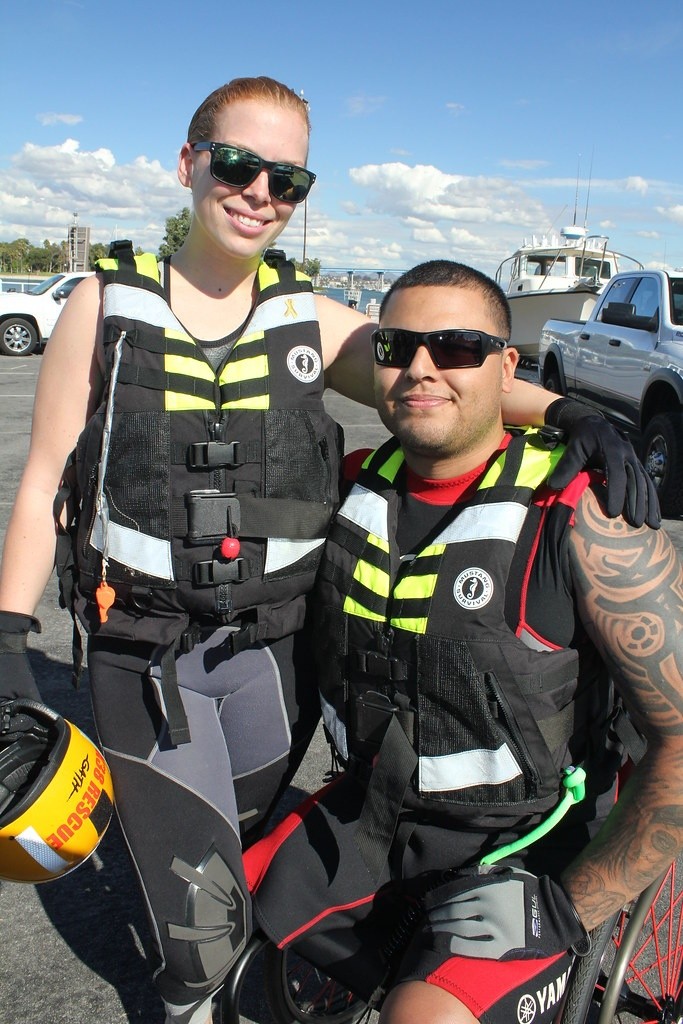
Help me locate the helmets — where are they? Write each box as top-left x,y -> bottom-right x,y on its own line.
0,698 -> 115,883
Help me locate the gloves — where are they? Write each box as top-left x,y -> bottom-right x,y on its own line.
0,610 -> 42,704
419,864 -> 593,961
545,397 -> 662,530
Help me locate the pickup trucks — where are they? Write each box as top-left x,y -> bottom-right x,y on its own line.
535,268 -> 682,522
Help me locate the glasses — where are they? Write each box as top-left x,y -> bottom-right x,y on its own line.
193,141 -> 316,203
369,328 -> 508,369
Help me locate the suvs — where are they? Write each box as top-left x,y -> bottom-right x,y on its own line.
0,270 -> 97,357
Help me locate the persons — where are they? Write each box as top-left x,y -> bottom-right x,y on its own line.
0,76 -> 662,1024
534,259 -> 552,276
236,259 -> 683,1024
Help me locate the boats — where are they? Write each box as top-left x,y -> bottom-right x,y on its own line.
492,142 -> 646,370
